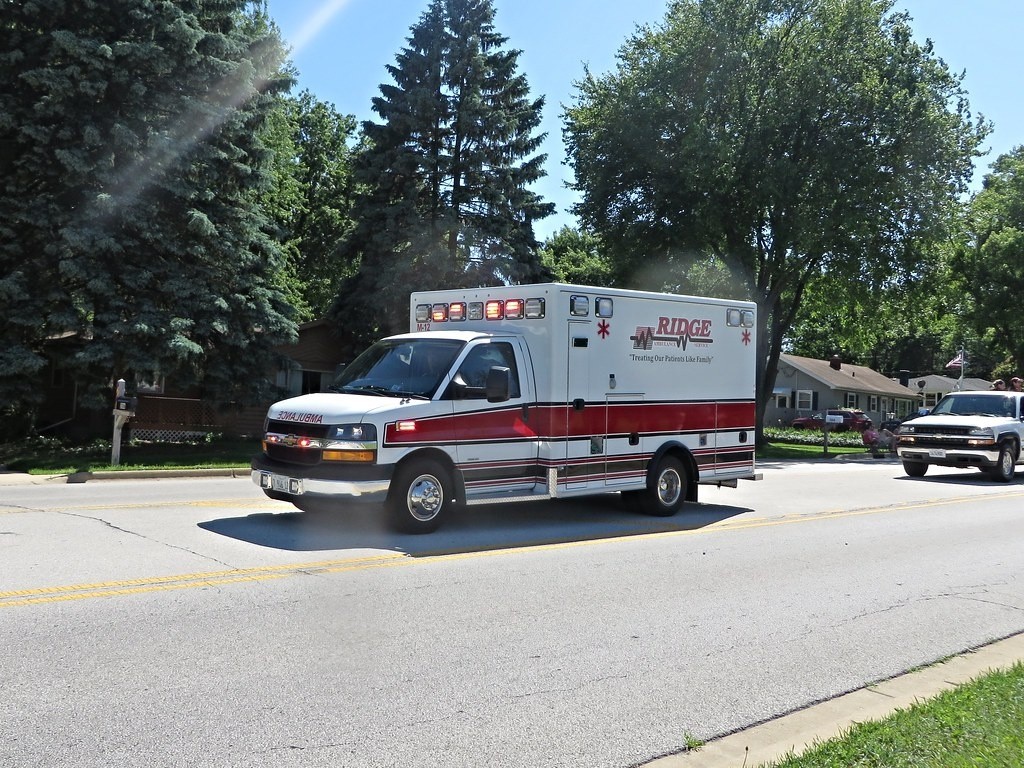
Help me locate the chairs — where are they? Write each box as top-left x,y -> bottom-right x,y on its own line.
857,421 -> 881,454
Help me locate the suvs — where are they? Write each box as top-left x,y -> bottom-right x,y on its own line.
880,390 -> 1024,482
790,406 -> 873,434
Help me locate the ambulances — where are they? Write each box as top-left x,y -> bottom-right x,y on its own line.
248,281 -> 760,538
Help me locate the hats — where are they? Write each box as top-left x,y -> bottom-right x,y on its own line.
1011,377 -> 1023,382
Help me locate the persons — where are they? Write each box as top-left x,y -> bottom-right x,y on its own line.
988,379 -> 1006,391
864,422 -> 899,452
1008,377 -> 1023,392
